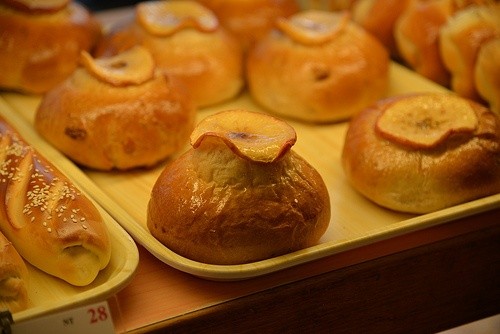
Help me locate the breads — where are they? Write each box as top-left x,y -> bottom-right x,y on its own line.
0,0 -> 500,309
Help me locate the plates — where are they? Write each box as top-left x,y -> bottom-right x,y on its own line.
0,59 -> 499,283
0,156 -> 141,322
